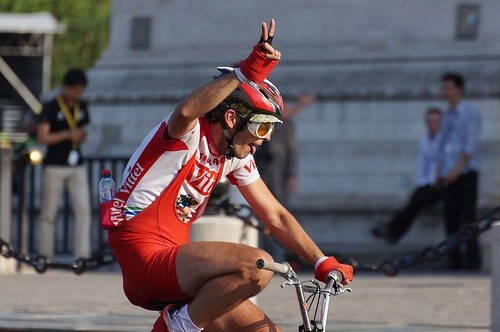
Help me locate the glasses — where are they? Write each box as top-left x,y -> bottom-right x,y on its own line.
236,115 -> 276,139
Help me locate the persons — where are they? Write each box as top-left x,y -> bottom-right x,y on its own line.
35,68 -> 90,263
251,95 -> 314,272
100,19 -> 353,332
370,73 -> 491,274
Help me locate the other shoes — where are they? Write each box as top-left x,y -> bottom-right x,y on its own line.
152,304 -> 180,332
372,225 -> 396,244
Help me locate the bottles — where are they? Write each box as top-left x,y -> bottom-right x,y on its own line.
98,169 -> 118,204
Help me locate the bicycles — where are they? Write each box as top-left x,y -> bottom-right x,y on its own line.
256,258 -> 352,332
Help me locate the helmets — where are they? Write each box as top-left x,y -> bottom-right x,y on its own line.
213,66 -> 283,160
